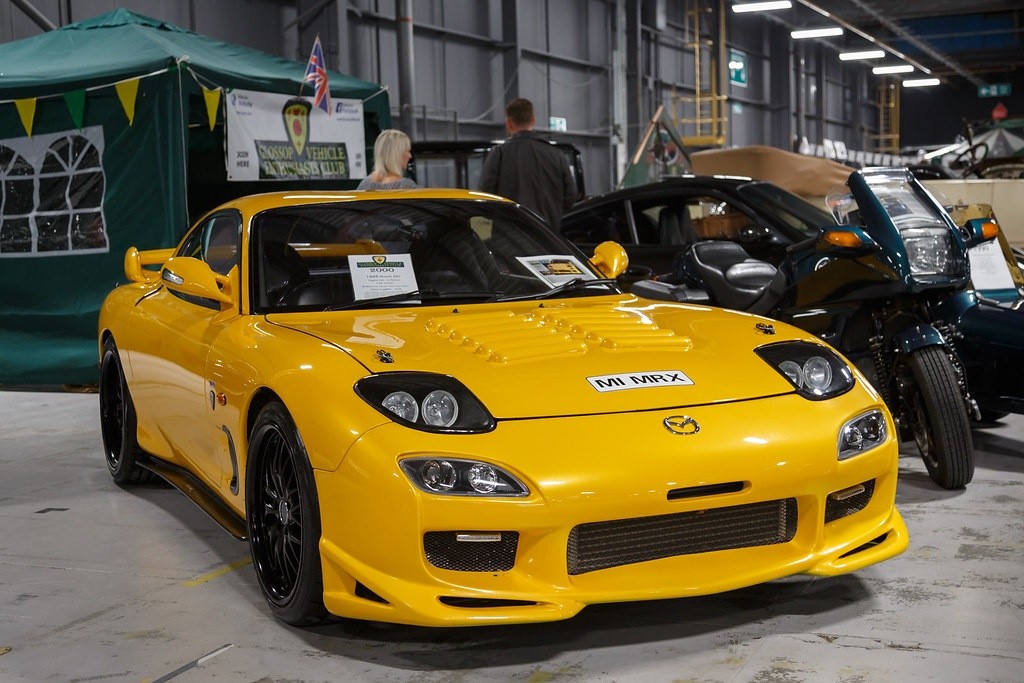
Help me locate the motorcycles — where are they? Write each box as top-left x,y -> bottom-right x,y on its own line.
608,165 -> 998,490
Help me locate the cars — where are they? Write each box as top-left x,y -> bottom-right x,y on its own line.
99,187 -> 913,628
543,174 -> 1022,425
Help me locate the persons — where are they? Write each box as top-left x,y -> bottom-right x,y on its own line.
357,129 -> 421,191
478,99 -> 578,239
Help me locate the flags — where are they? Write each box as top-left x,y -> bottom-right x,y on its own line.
304,38 -> 332,116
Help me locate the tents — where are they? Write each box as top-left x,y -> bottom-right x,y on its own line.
0,8 -> 391,389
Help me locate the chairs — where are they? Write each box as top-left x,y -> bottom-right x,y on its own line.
231,238 -> 310,308
408,239 -> 489,295
659,202 -> 697,244
